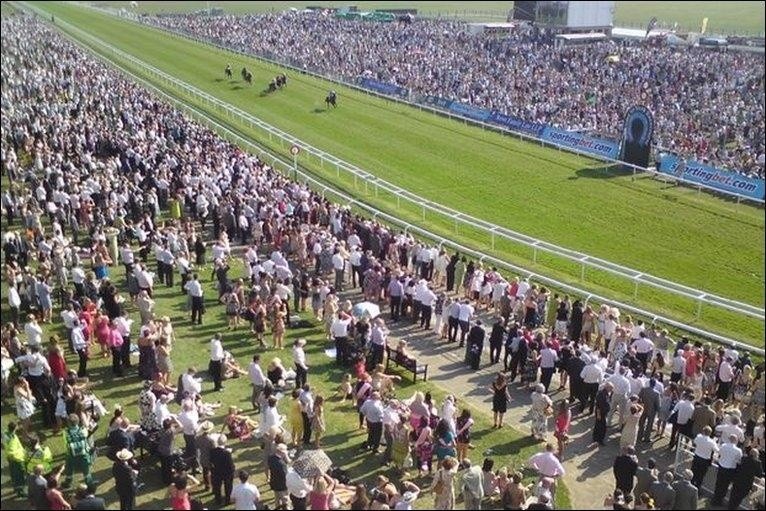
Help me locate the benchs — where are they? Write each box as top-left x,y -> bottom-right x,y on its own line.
380,340 -> 430,387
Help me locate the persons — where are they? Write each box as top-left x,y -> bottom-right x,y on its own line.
0,0 -> 765,510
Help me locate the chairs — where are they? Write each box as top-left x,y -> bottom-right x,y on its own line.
61,398 -> 95,417
